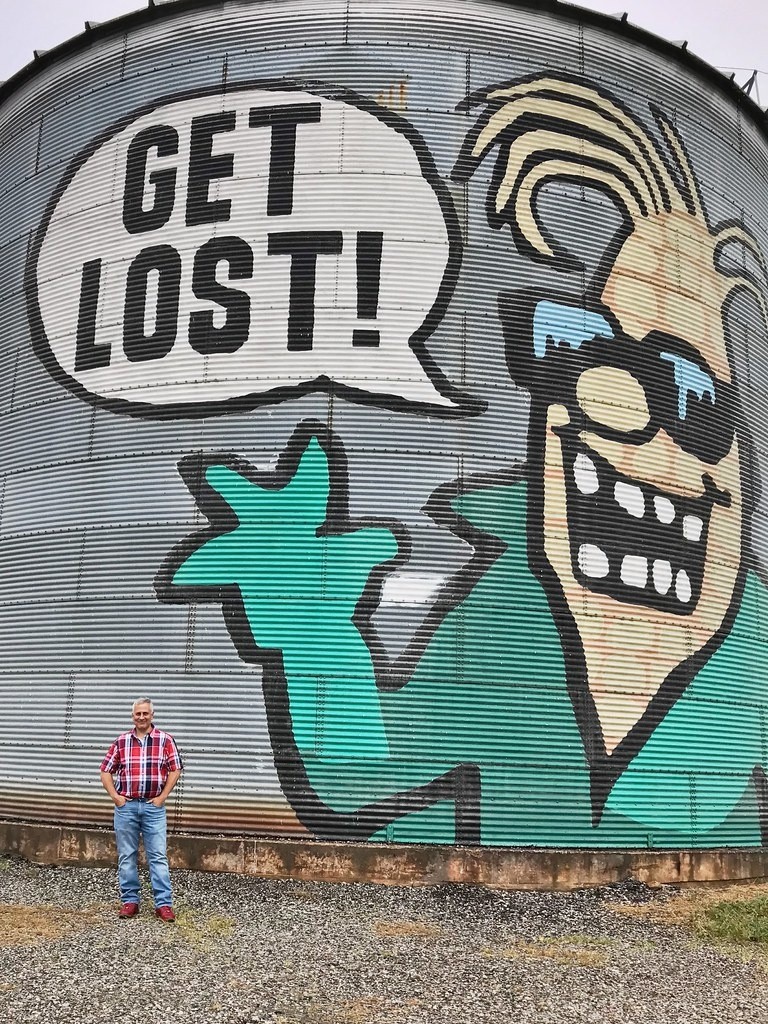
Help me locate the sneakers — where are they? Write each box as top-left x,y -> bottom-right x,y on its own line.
155,906 -> 175,922
118,903 -> 140,917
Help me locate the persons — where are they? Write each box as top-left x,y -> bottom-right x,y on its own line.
98,697 -> 183,921
